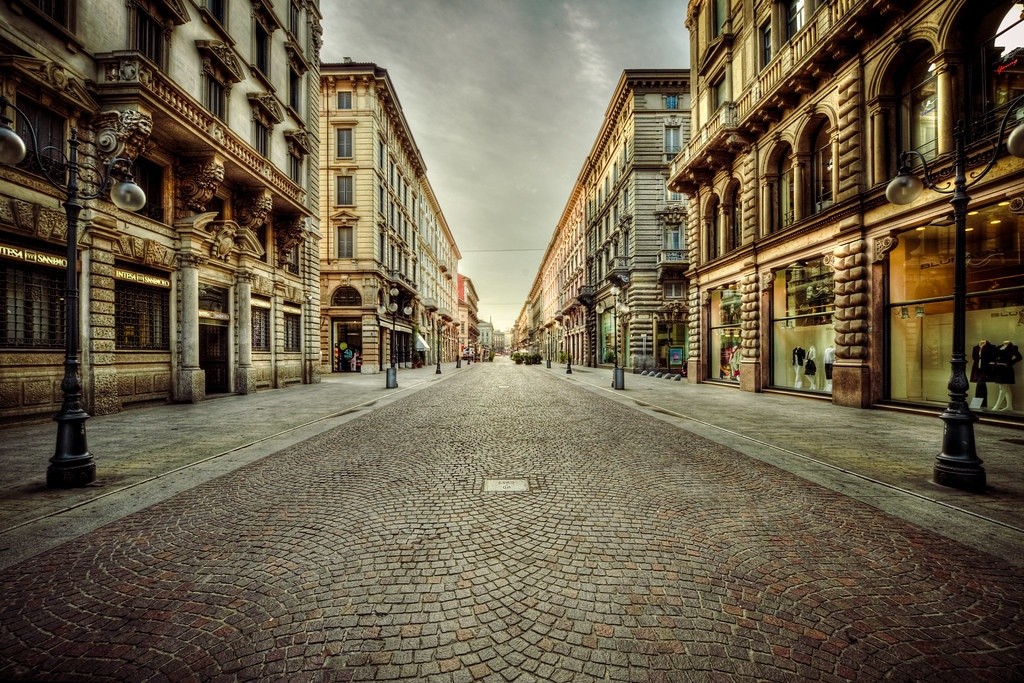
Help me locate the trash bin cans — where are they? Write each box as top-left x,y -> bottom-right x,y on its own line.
385,367 -> 398,388
547,360 -> 551,368
614,368 -> 625,390
457,360 -> 462,368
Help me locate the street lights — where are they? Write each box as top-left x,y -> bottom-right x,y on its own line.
596,281 -> 629,390
386,284 -> 412,389
0,96 -> 146,489
556,313 -> 580,374
427,318 -> 446,374
885,94 -> 1024,494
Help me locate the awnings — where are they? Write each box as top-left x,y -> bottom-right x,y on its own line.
416,334 -> 431,351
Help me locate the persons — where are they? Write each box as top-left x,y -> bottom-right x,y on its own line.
729,345 -> 743,378
970,341 -> 1022,411
792,345 -> 836,392
